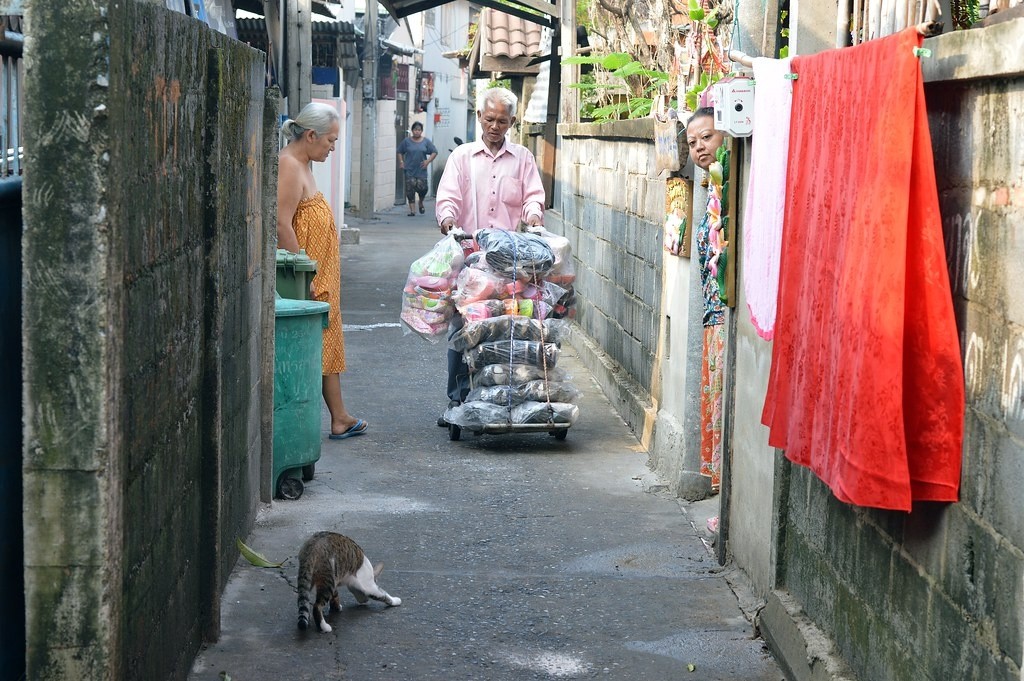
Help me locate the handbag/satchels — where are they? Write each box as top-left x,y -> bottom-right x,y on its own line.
400,224 -> 468,344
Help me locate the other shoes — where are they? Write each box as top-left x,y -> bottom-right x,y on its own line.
407,212 -> 414,216
420,204 -> 425,214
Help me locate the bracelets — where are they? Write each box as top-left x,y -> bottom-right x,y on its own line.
399,160 -> 404,163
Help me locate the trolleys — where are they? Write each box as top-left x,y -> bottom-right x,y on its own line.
445,234 -> 571,442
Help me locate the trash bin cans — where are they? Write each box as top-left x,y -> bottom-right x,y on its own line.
271,300 -> 330,496
275,244 -> 319,300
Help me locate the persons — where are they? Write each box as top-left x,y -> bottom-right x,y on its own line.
686,107 -> 724,529
436,88 -> 546,427
397,122 -> 438,216
276,102 -> 370,440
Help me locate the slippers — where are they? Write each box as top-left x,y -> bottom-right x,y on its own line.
329,419 -> 369,439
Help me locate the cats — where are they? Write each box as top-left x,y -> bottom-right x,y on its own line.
297,531 -> 402,633
